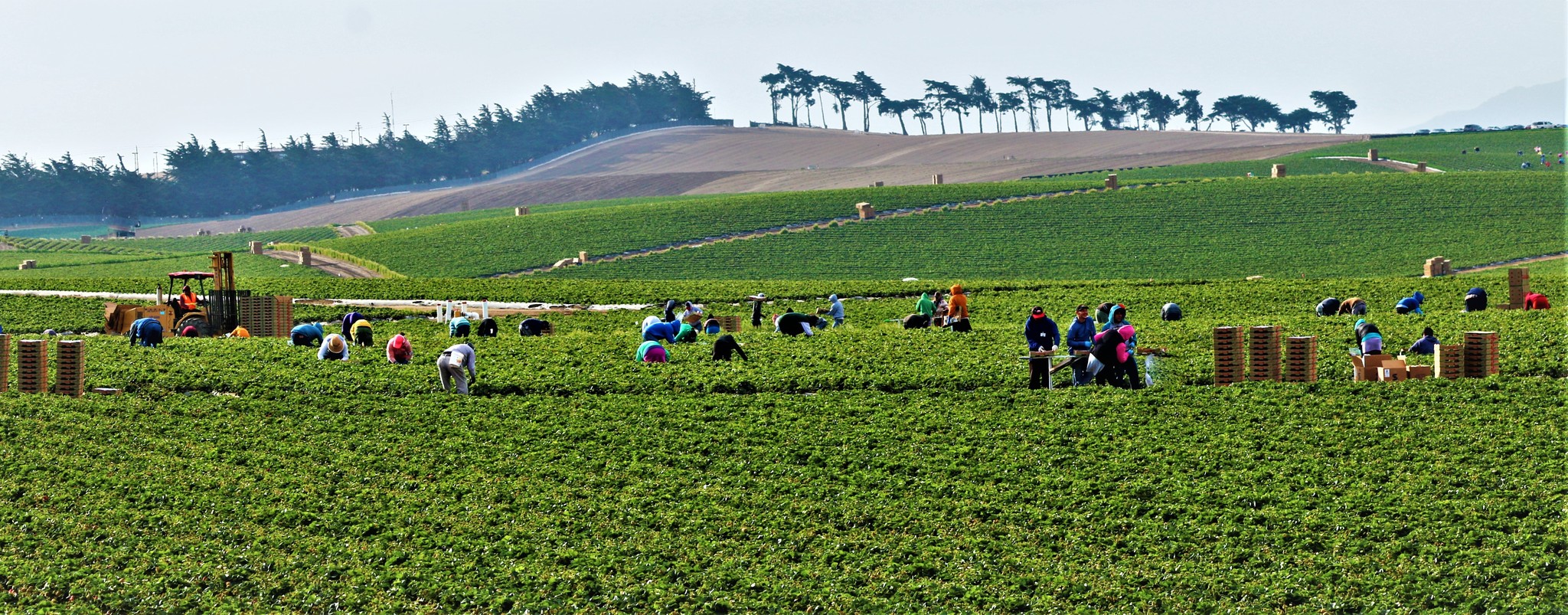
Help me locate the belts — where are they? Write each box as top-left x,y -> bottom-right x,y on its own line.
443,354 -> 451,356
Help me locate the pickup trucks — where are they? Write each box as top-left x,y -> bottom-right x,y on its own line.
1530,121 -> 1553,130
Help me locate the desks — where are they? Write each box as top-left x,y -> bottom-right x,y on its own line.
1018,356 -> 1088,390
883,316 -> 943,329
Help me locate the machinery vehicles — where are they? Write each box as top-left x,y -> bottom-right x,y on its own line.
119,250 -> 251,338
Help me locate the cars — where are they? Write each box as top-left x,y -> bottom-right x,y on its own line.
1429,129 -> 1446,134
1554,124 -> 1568,128
1511,125 -> 1525,130
1413,129 -> 1430,134
1524,124 -> 1531,130
1500,125 -> 1513,131
1484,126 -> 1501,131
1448,128 -> 1464,132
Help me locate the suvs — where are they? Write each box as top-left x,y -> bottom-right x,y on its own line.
1463,124 -> 1484,132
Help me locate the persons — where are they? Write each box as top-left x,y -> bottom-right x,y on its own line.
181,326 -> 201,338
181,286 -> 208,315
904,284 -> 974,333
228,326 -> 250,337
1315,288 -> 1550,354
129,318 -> 162,348
1023,302 -> 1183,389
634,294 -> 844,363
1462,147 -> 1568,169
289,311 -> 555,365
438,343 -> 476,395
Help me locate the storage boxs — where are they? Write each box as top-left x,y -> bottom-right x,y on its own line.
1105,174 -> 1118,190
553,251 -> 587,268
1414,162 -> 1426,173
1271,164 -> 1286,178
240,295 -> 293,337
1368,149 -> 1379,161
542,324 -> 556,336
1350,355 -> 1431,383
515,206 -> 529,217
1423,256 -> 1451,277
1213,326 -> 1317,387
1434,331 -> 1499,382
1495,267 -> 1529,311
714,316 -> 742,332
298,245 -> 311,267
268,241 -> 280,245
932,174 -> 944,184
868,181 -> 883,188
18,260 -> 36,271
856,202 -> 875,220
0,334 -> 121,395
249,240 -> 262,255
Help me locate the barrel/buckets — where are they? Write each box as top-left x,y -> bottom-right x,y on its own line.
449,351 -> 464,368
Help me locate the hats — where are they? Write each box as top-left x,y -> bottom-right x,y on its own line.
1076,304 -> 1089,311
674,303 -> 677,306
1422,327 -> 1433,335
758,293 -> 764,297
543,320 -> 550,330
394,334 -> 404,348
328,337 -> 344,353
183,286 -> 190,291
685,303 -> 689,308
1032,306 -> 1045,318
1317,306 -> 1323,316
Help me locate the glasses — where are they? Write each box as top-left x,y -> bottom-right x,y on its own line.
1078,305 -> 1086,310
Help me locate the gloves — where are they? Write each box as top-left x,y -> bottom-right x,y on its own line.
1052,345 -> 1058,351
1127,350 -> 1134,355
947,320 -> 951,324
1129,342 -> 1135,348
1039,346 -> 1045,352
467,377 -> 476,387
1085,341 -> 1093,348
